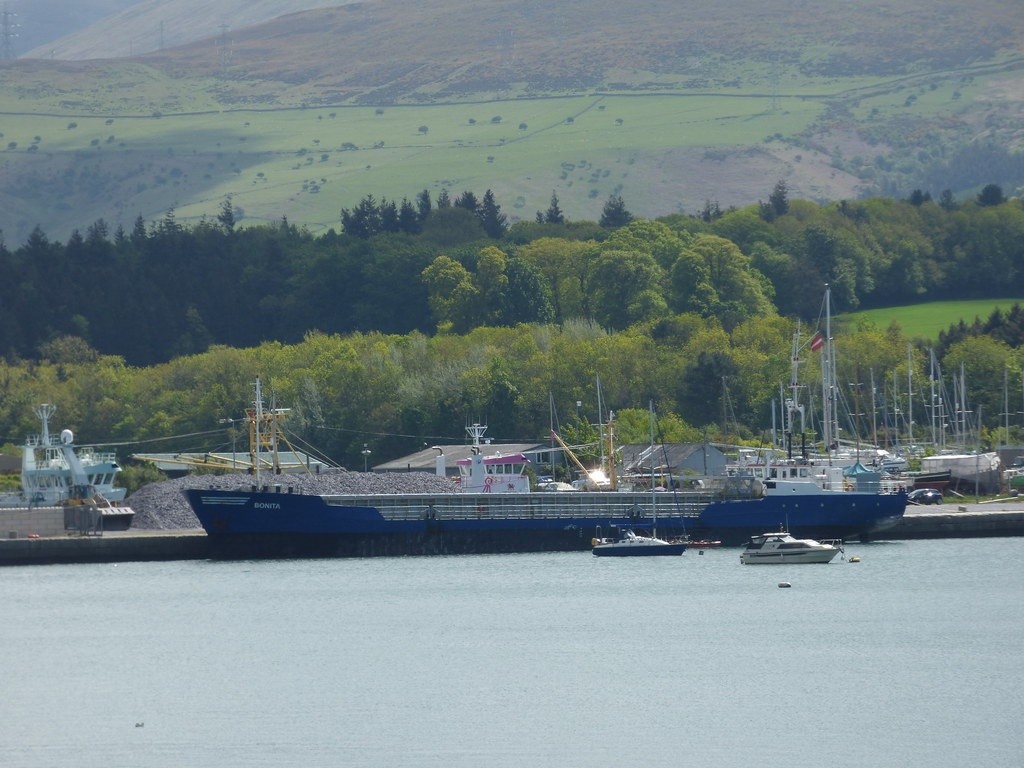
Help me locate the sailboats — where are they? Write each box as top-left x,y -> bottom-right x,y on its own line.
738,283 -> 1023,491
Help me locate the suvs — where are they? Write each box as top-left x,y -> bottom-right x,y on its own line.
578,472 -> 610,490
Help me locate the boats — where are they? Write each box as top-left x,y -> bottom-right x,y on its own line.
130,377 -> 911,553
0,404 -> 128,507
739,528 -> 839,565
592,525 -> 685,558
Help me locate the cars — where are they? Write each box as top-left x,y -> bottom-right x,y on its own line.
907,489 -> 944,506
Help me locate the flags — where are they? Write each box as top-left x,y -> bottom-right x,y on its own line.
811,334 -> 824,352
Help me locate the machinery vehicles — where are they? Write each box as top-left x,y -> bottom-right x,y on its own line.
56,484 -> 135,532
